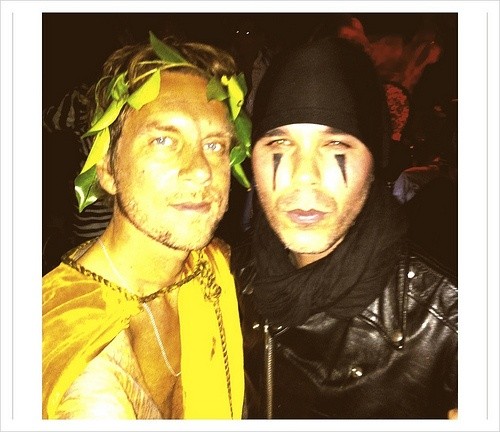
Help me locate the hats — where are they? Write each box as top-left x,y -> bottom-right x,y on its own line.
250,40 -> 390,170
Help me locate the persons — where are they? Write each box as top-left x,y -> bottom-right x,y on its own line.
43,46 -> 115,246
43,25 -> 247,421
230,33 -> 457,419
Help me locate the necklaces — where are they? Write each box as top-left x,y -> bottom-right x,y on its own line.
94,233 -> 184,379
